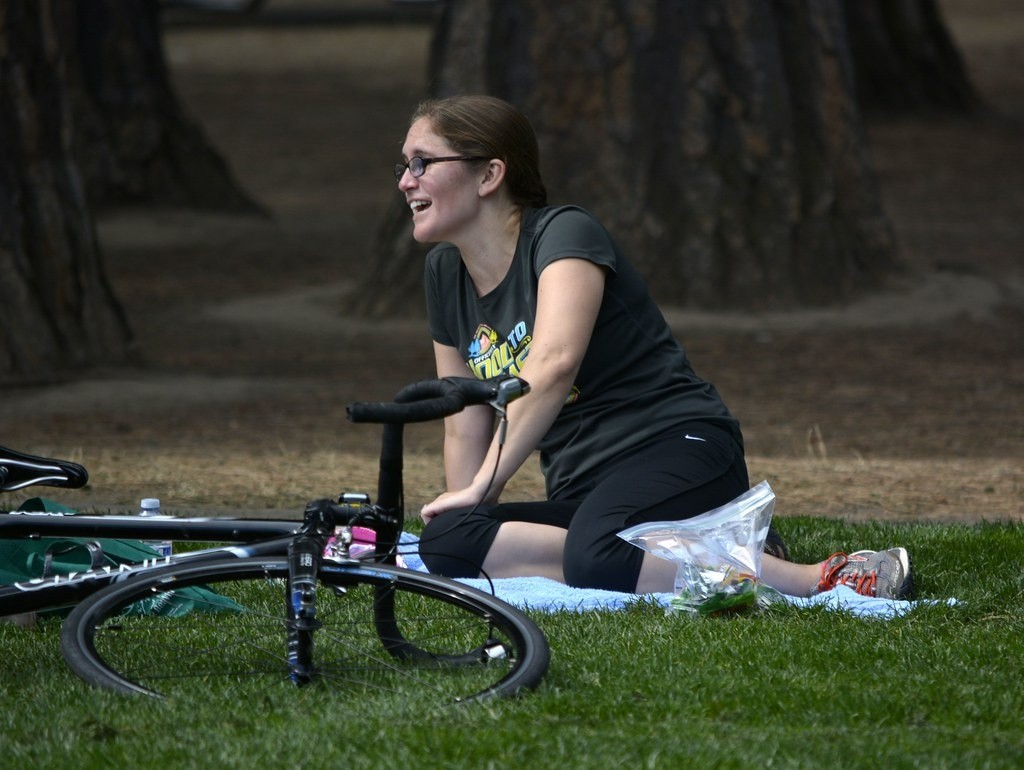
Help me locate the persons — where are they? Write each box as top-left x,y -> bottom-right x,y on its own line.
397,97 -> 915,597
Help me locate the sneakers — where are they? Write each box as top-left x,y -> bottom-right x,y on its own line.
762,527 -> 786,562
810,548 -> 909,598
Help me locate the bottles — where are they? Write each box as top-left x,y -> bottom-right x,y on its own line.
137,498 -> 174,557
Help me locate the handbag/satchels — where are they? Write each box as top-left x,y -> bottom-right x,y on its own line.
0,496 -> 248,617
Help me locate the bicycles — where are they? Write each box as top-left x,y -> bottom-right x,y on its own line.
0,373 -> 550,705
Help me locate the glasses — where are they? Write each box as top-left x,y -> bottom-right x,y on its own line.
394,154 -> 488,184
